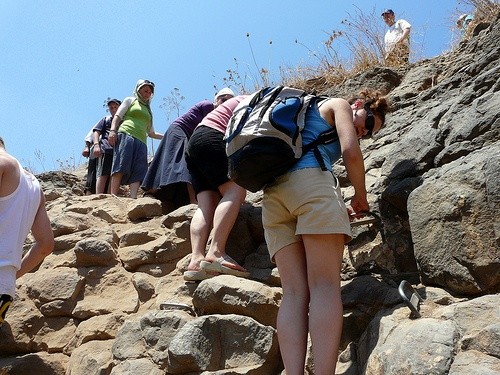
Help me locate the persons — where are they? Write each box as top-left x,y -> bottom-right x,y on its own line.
262,89 -> 389,375
82,117 -> 104,196
183,95 -> 251,280
0,137 -> 54,327
381,9 -> 411,62
107,79 -> 164,199
140,87 -> 235,204
457,14 -> 475,41
93,99 -> 121,194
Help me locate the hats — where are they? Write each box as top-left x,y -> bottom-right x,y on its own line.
381,9 -> 395,16
107,99 -> 122,106
214,87 -> 235,100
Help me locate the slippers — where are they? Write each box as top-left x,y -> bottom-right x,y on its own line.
182,255 -> 250,281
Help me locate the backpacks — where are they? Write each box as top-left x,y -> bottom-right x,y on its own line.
224,85 -> 339,192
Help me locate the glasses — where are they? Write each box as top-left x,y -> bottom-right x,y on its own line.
362,108 -> 375,139
138,80 -> 155,86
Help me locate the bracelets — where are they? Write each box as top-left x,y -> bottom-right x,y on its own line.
110,129 -> 117,132
94,142 -> 99,145
85,145 -> 89,147
